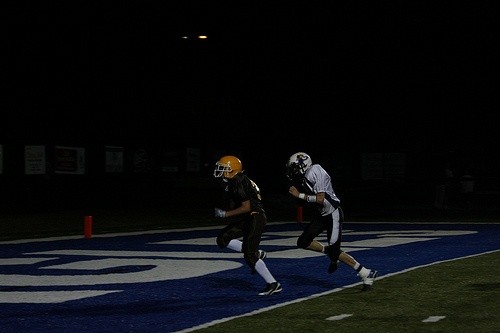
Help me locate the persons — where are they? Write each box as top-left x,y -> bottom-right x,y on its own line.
214,156 -> 282,295
287,152 -> 379,291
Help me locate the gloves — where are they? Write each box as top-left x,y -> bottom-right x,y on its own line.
215,208 -> 226,218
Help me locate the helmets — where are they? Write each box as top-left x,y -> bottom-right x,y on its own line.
286,152 -> 312,174
215,155 -> 242,182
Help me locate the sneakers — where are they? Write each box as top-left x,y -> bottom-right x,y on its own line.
259,248 -> 267,260
328,252 -> 340,274
258,282 -> 283,296
361,269 -> 378,291
